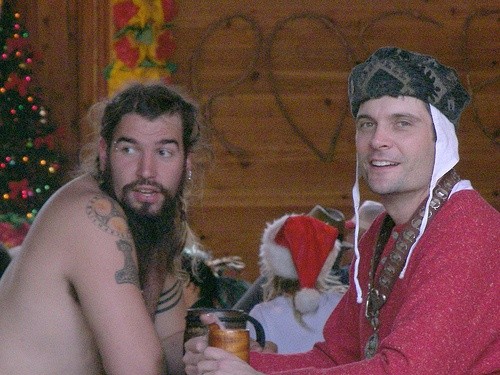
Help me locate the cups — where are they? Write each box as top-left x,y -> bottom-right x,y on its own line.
207,309 -> 265,366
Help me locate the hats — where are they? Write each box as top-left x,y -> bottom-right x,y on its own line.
259,213 -> 340,315
346,46 -> 471,123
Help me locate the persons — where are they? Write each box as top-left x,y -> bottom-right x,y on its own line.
246,212 -> 350,354
183,45 -> 500,375
231,204 -> 349,323
180,251 -> 216,357
0,81 -> 278,374
